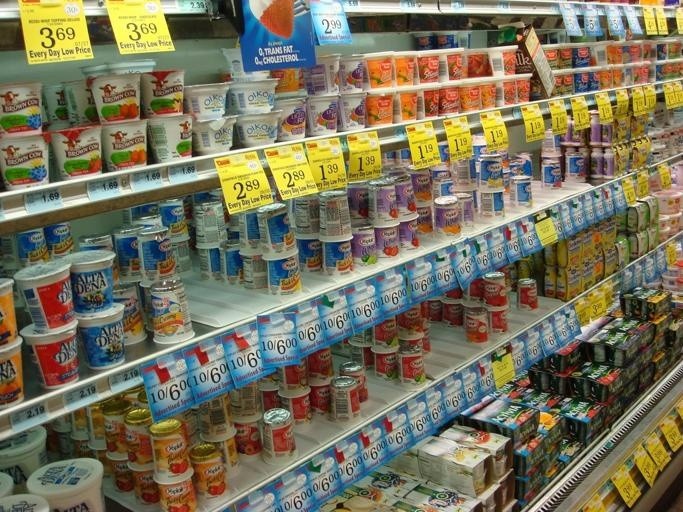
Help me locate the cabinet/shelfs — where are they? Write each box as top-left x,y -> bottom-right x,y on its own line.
0,0 -> 683,512
